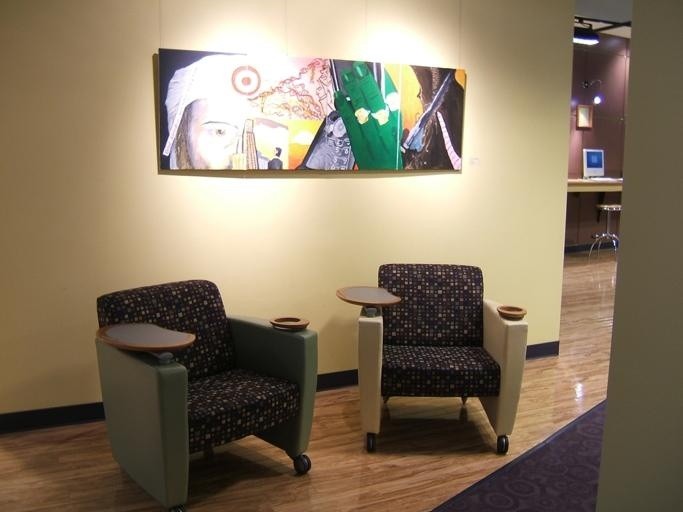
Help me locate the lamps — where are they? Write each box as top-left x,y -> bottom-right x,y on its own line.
573,19 -> 600,46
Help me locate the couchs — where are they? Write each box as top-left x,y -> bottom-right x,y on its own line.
337,263 -> 529,456
96,279 -> 319,512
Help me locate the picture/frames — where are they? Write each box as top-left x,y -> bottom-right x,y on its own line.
158,48 -> 466,172
576,104 -> 593,129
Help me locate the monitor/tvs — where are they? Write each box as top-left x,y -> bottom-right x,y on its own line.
583,149 -> 604,178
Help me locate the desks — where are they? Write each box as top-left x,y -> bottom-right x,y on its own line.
566,177 -> 623,193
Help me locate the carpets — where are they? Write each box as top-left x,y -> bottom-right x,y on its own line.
427,398 -> 607,512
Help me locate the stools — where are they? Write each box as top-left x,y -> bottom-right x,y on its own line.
587,204 -> 621,263
